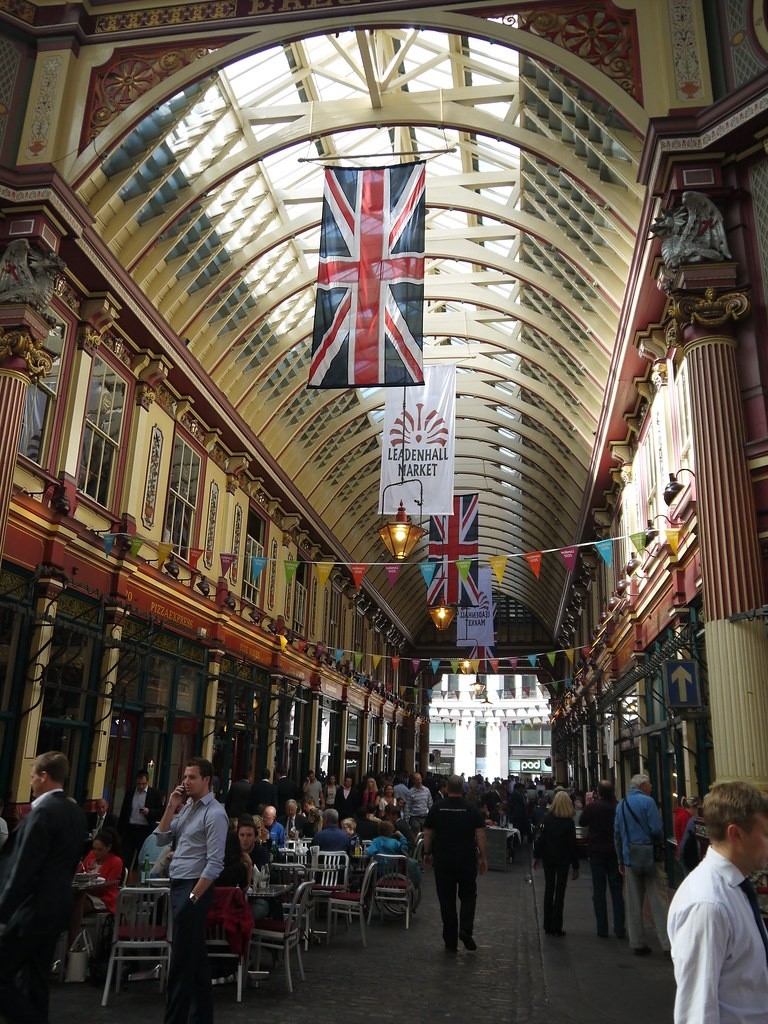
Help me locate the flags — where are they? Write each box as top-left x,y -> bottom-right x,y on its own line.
466,603 -> 497,673
456,565 -> 494,646
379,365 -> 455,516
427,494 -> 479,606
306,161 -> 427,388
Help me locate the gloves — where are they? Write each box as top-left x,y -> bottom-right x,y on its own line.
572,869 -> 579,880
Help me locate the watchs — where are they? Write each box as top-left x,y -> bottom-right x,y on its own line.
189,893 -> 198,902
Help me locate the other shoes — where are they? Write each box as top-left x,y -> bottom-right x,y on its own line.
445,943 -> 458,952
664,952 -> 671,956
617,932 -> 625,938
636,946 -> 652,956
459,931 -> 476,951
598,929 -> 608,937
546,929 -> 566,936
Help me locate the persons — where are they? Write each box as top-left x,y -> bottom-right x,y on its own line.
153,758 -> 229,1024
0,752 -> 87,1024
613,774 -> 671,955
423,776 -> 488,952
579,781 -> 626,938
667,783 -> 768,1024
70,770 -> 175,978
533,791 -> 580,936
674,797 -> 710,875
211,765 -> 448,985
464,775 -> 598,855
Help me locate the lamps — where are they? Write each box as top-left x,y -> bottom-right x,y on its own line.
640,514 -> 684,548
377,262 -> 430,560
546,566 -> 648,725
663,468 -> 694,506
90,521 -> 132,550
626,548 -> 656,576
426,514 -> 460,632
179,570 -> 431,724
456,609 -> 492,711
145,552 -> 180,579
23,483 -> 71,517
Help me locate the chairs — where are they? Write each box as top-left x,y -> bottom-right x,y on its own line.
102,832 -> 424,1008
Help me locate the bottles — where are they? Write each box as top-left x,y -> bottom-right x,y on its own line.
258,864 -> 271,889
355,837 -> 360,855
141,853 -> 151,885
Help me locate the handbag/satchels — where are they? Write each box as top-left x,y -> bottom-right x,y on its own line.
628,843 -> 657,873
64,929 -> 92,983
653,844 -> 667,862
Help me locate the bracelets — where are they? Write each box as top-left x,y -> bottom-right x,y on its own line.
424,851 -> 432,855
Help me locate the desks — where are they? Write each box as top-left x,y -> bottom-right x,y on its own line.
62,881 -> 117,974
120,885 -> 293,982
483,828 -> 521,870
286,864 -> 346,942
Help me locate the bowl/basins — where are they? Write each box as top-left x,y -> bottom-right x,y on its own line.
145,879 -> 169,886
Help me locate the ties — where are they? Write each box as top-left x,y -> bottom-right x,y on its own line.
501,817 -> 503,824
288,818 -> 293,834
738,878 -> 768,969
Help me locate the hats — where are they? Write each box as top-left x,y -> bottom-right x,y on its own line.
555,786 -> 564,791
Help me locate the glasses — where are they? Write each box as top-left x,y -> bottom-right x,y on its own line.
342,826 -> 349,830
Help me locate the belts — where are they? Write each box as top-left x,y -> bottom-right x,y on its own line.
416,816 -> 427,819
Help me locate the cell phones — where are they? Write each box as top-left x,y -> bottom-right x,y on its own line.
182,783 -> 186,801
139,809 -> 144,813
